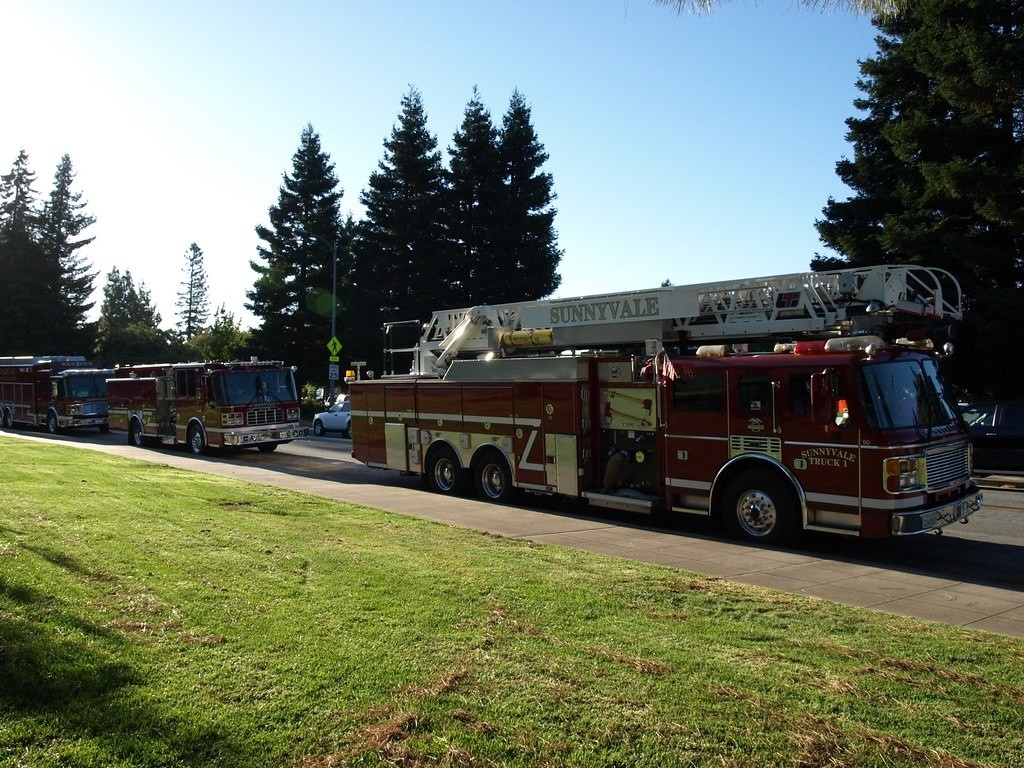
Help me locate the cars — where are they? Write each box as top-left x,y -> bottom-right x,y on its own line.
951,398 -> 1024,481
312,400 -> 353,439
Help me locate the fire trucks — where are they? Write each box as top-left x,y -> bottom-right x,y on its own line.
348,260 -> 984,546
0,353 -> 115,434
106,356 -> 310,455
